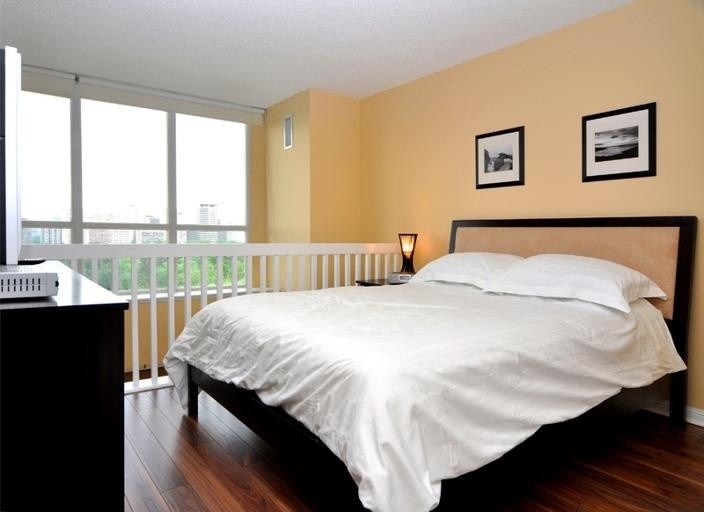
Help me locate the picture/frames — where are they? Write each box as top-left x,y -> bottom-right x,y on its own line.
582,102 -> 657,181
475,125 -> 525,189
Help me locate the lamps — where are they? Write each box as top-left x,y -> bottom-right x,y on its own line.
398,232 -> 418,274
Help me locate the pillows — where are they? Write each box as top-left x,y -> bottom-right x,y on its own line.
484,253 -> 668,313
409,252 -> 525,288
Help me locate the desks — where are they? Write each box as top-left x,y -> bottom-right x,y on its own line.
0,260 -> 129,510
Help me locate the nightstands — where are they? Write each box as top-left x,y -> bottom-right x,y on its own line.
356,278 -> 394,285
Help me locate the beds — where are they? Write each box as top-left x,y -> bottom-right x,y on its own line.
161,216 -> 697,512
